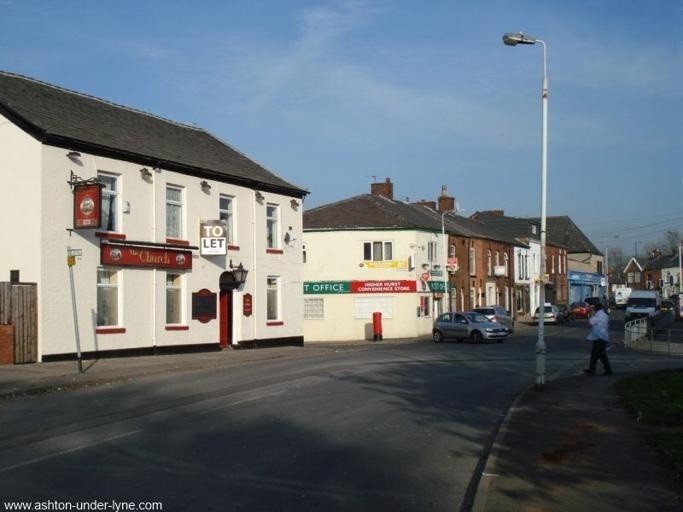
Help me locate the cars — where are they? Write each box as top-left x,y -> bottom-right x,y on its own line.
661,298 -> 677,315
473,306 -> 516,332
533,297 -> 609,323
432,310 -> 509,344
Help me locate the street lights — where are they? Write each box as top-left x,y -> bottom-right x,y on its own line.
502,30 -> 550,393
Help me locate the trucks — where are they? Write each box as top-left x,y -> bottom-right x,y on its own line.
614,287 -> 634,308
625,290 -> 662,325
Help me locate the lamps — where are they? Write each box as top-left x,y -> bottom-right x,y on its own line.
229,259 -> 248,284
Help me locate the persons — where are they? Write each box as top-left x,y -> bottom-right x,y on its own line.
580,302 -> 615,376
